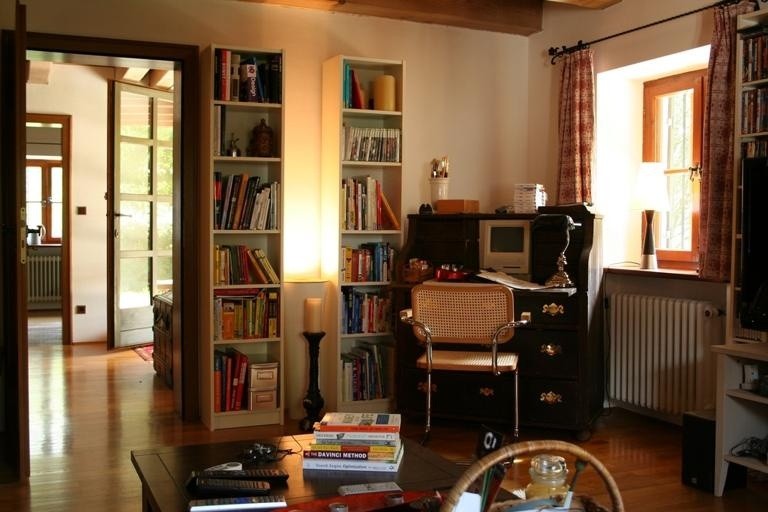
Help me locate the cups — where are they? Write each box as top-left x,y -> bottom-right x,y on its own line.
428,177 -> 448,205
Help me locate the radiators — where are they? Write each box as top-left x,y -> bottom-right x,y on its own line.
27,255 -> 61,303
608,290 -> 719,417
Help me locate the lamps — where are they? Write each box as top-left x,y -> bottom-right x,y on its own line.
528,214 -> 582,288
628,161 -> 674,269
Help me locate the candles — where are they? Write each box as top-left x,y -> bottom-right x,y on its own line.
304,297 -> 322,333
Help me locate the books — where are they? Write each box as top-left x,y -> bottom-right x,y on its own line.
213,47 -> 282,105
742,35 -> 768,158
344,65 -> 365,109
215,105 -> 225,157
340,339 -> 388,401
341,242 -> 399,282
302,412 -> 404,472
214,289 -> 280,340
341,176 -> 399,231
213,348 -> 248,413
214,172 -> 281,231
341,286 -> 396,335
343,126 -> 400,162
215,245 -> 280,284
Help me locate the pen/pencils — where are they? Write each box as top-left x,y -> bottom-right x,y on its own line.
430,155 -> 449,179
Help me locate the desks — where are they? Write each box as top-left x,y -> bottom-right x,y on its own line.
131,433 -> 522,512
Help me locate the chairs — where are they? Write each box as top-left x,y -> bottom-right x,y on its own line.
439,441 -> 624,512
400,282 -> 533,445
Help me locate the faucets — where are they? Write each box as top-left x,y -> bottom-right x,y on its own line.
26,225 -> 42,236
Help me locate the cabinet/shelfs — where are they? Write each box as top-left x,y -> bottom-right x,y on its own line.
153,290 -> 173,387
397,209 -> 603,434
199,42 -> 287,432
322,56 -> 406,413
711,11 -> 768,506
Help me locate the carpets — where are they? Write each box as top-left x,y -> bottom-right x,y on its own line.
132,345 -> 155,361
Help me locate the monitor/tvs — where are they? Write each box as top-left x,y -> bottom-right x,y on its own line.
479,220 -> 530,275
739,156 -> 768,330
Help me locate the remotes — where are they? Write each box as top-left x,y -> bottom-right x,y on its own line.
194,478 -> 271,492
192,468 -> 289,481
203,461 -> 243,471
187,495 -> 288,512
336,481 -> 404,496
